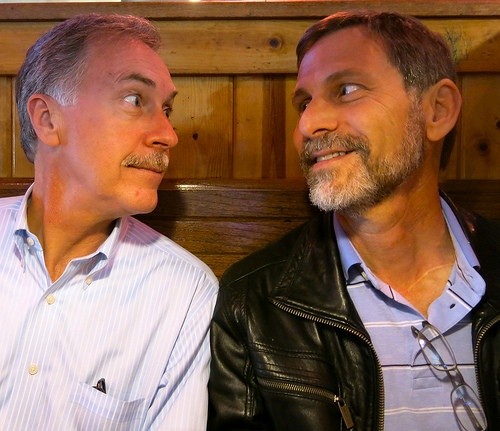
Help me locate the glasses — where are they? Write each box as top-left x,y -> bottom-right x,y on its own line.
413,321 -> 489,431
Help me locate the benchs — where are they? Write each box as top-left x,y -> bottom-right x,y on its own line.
0,178 -> 500,285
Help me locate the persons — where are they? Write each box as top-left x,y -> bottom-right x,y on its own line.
3,8 -> 221,430
206,8 -> 498,431
